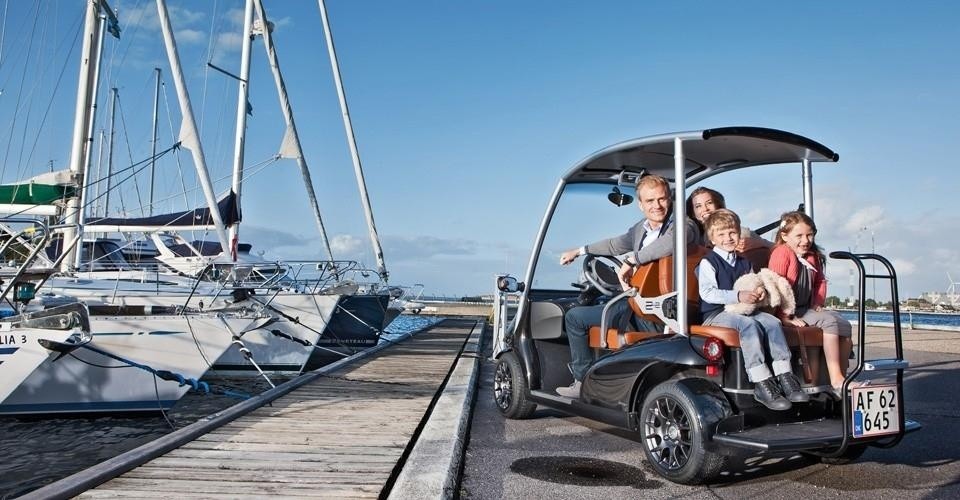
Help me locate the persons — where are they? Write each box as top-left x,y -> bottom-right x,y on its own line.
686,186 -> 777,252
555,174 -> 701,402
768,210 -> 873,394
692,206 -> 810,411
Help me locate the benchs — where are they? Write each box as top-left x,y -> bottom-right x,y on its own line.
588,243 -> 853,390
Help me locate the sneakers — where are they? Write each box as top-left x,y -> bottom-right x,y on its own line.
775,371 -> 811,404
753,377 -> 793,412
555,381 -> 581,399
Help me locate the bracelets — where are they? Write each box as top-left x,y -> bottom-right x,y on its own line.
620,254 -> 636,269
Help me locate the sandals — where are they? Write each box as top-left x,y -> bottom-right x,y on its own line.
832,379 -> 872,399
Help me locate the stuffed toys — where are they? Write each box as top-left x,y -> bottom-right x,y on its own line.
724,266 -> 797,319
